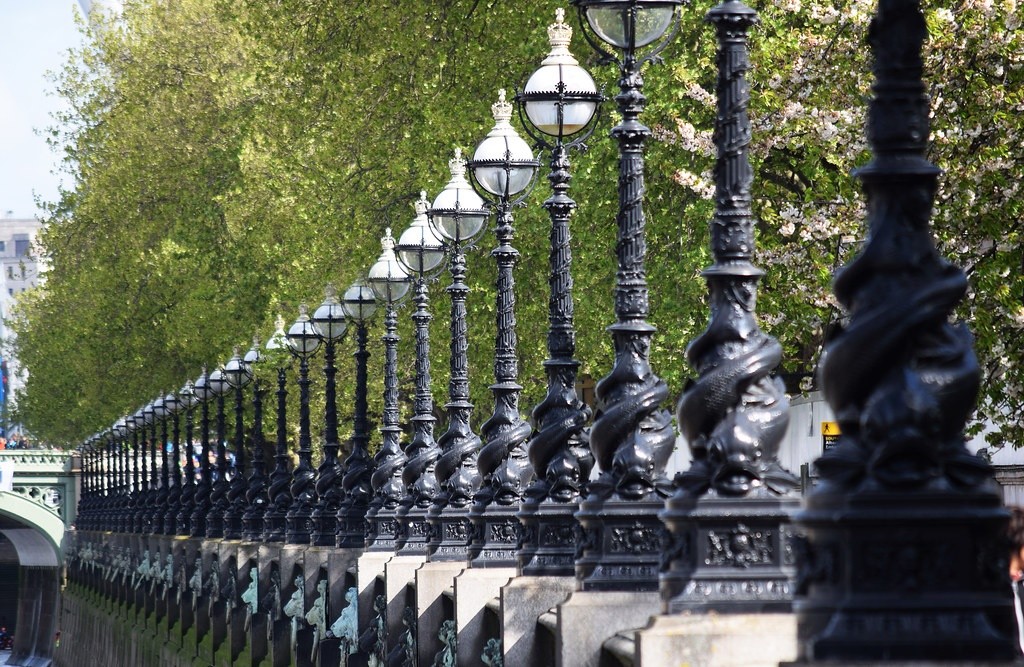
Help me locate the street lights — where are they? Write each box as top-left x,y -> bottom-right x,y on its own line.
306,289 -> 351,547
459,87 -> 540,570
75,303 -> 322,545
335,274 -> 379,550
573,1 -> 689,591
510,5 -> 607,584
363,226 -> 417,554
391,190 -> 455,560
423,146 -> 496,569
